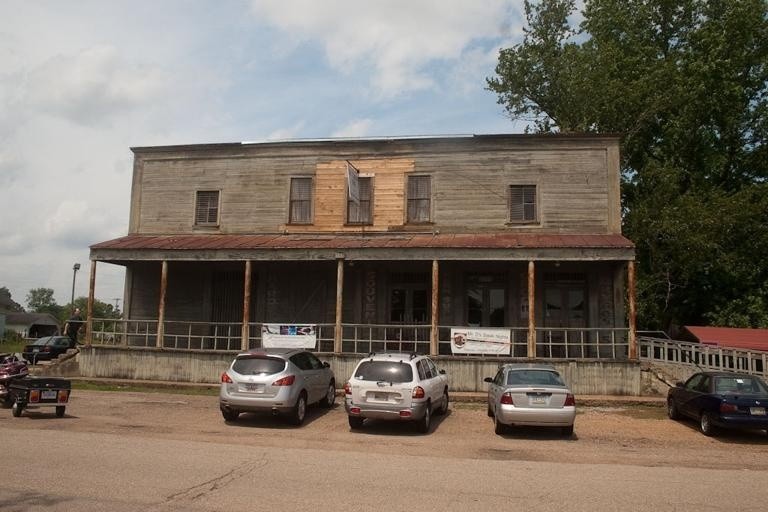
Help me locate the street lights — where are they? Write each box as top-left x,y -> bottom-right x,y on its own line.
70,264 -> 80,316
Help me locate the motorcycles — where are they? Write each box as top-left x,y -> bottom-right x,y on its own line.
0,352 -> 71,417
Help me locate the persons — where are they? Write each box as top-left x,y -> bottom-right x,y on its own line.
63,308 -> 83,348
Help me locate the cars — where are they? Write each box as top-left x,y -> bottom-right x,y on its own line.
667,371 -> 767,436
22,336 -> 84,363
